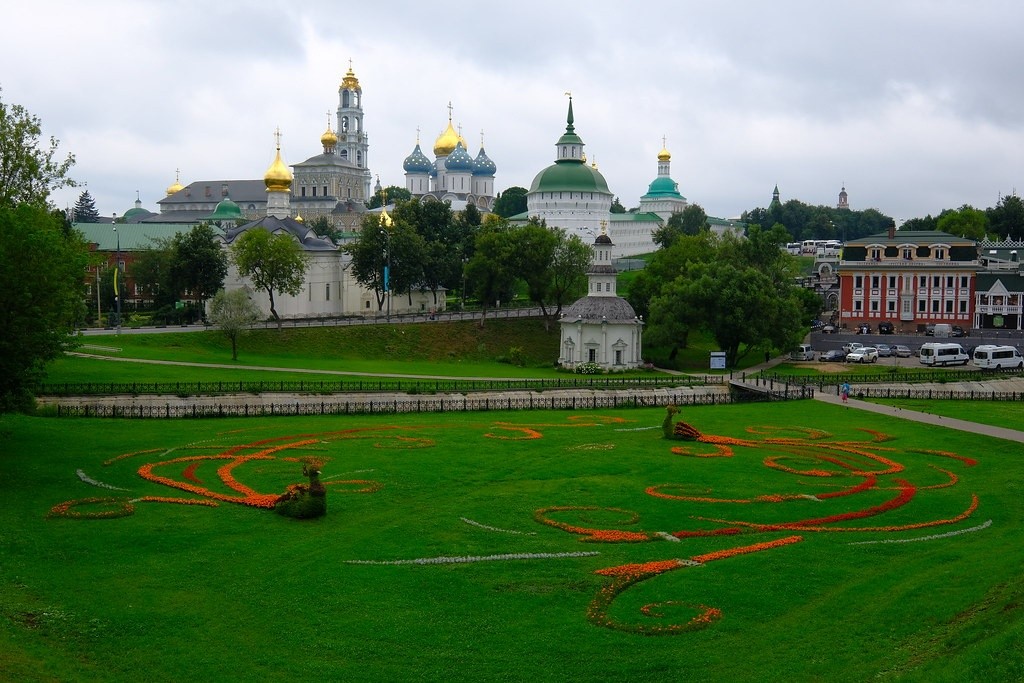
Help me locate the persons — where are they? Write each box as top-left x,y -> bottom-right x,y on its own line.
765,351 -> 769,363
841,379 -> 850,396
842,390 -> 848,402
814,319 -> 823,326
862,326 -> 867,334
854,325 -> 861,335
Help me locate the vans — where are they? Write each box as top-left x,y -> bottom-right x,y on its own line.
972,344 -> 1024,372
790,343 -> 816,361
918,342 -> 970,367
786,238 -> 844,257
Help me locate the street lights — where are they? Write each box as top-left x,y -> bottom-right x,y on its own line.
377,223 -> 391,322
111,220 -> 121,325
462,254 -> 468,303
582,226 -> 597,242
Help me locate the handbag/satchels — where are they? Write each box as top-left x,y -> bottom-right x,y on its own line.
842,384 -> 847,392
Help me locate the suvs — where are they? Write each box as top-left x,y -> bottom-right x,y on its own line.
842,342 -> 863,353
846,346 -> 879,364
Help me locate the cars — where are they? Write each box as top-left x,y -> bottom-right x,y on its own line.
821,325 -> 837,334
871,343 -> 891,357
818,349 -> 846,362
890,344 -> 912,357
914,346 -> 922,357
857,323 -> 871,334
952,326 -> 964,337
966,346 -> 979,359
925,325 -> 935,335
